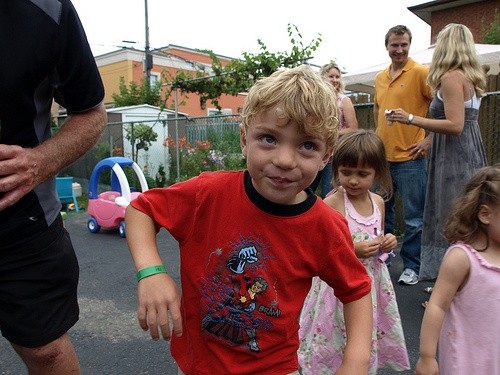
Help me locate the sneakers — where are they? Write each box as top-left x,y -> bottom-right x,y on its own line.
397,267 -> 418,286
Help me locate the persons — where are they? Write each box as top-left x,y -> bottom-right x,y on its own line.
385,22 -> 487,308
310,63 -> 359,199
373,25 -> 436,284
314,129 -> 397,375
0,0 -> 106,375
124,63 -> 374,375
414,166 -> 500,375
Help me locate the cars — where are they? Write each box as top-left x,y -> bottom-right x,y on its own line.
88,157 -> 149,238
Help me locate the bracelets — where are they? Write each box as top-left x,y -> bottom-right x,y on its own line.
135,264 -> 168,282
386,249 -> 396,258
407,113 -> 414,124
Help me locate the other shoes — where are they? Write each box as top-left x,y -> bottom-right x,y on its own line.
421,301 -> 428,309
423,286 -> 434,294
